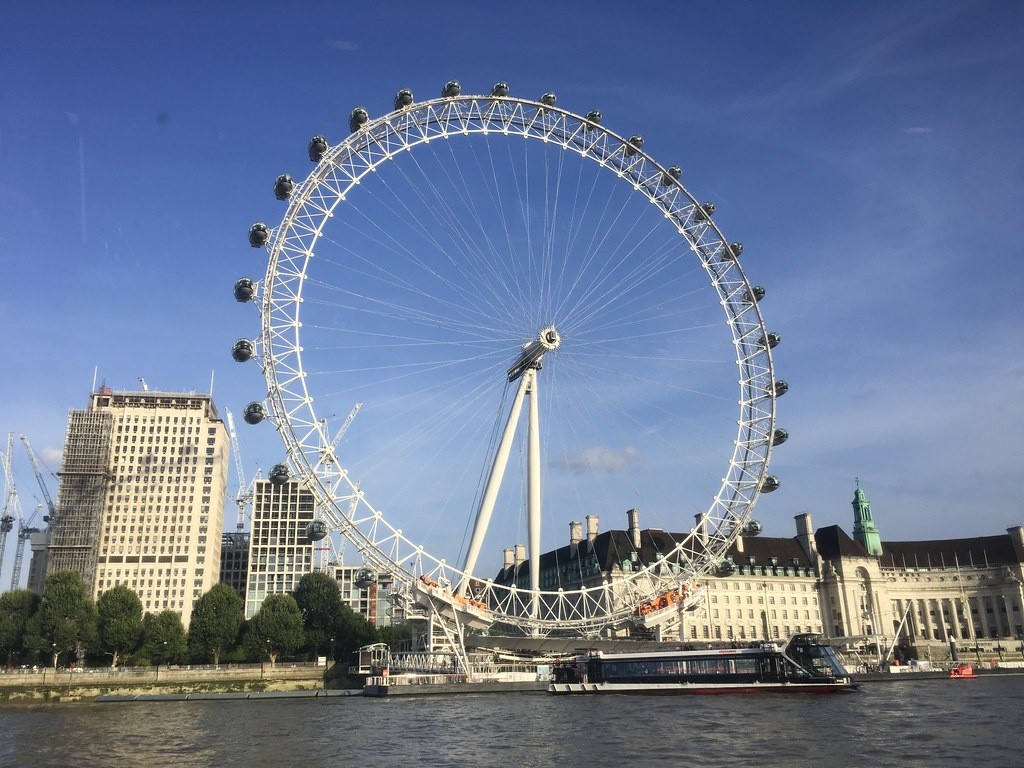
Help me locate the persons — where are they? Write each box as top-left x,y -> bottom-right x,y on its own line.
732,640 -> 765,649
707,643 -> 712,650
553,658 -> 577,683
676,643 -> 694,651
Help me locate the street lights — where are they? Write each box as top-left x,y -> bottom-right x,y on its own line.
155,642 -> 168,683
260,637 -> 270,677
326,633 -> 334,671
974,633 -> 980,662
1020,634 -> 1024,661
43,643 -> 57,688
996,634 -> 1003,662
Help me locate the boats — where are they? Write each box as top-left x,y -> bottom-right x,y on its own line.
413,575 -> 494,633
948,663 -> 978,679
363,646 -> 578,698
546,631 -> 862,693
786,603 -> 949,682
630,580 -> 707,631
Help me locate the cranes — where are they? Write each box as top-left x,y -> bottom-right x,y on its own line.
0,431 -> 11,575
318,399 -> 362,579
0,451 -> 43,596
17,429 -> 58,524
225,488 -> 252,519
225,407 -> 261,536
328,480 -> 361,566
